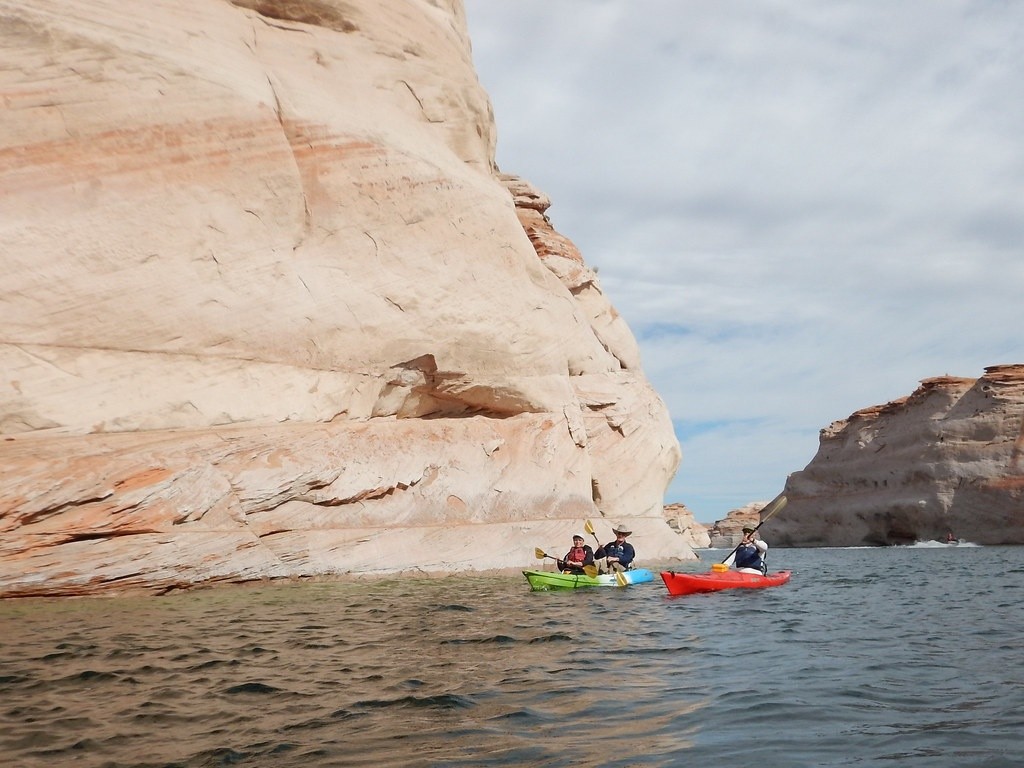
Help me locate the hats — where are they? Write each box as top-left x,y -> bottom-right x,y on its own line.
742,524 -> 755,531
612,525 -> 632,537
573,534 -> 584,540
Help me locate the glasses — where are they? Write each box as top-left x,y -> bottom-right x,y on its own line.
743,531 -> 750,533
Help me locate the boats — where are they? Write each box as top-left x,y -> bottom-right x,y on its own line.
523,569 -> 654,591
659,568 -> 791,596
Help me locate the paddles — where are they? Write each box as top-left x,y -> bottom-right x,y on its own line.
722,496 -> 788,564
534,547 -> 597,579
584,519 -> 628,586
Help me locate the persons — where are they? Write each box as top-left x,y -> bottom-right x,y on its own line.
594,525 -> 635,574
555,533 -> 596,575
723,523 -> 768,575
947,533 -> 954,541
